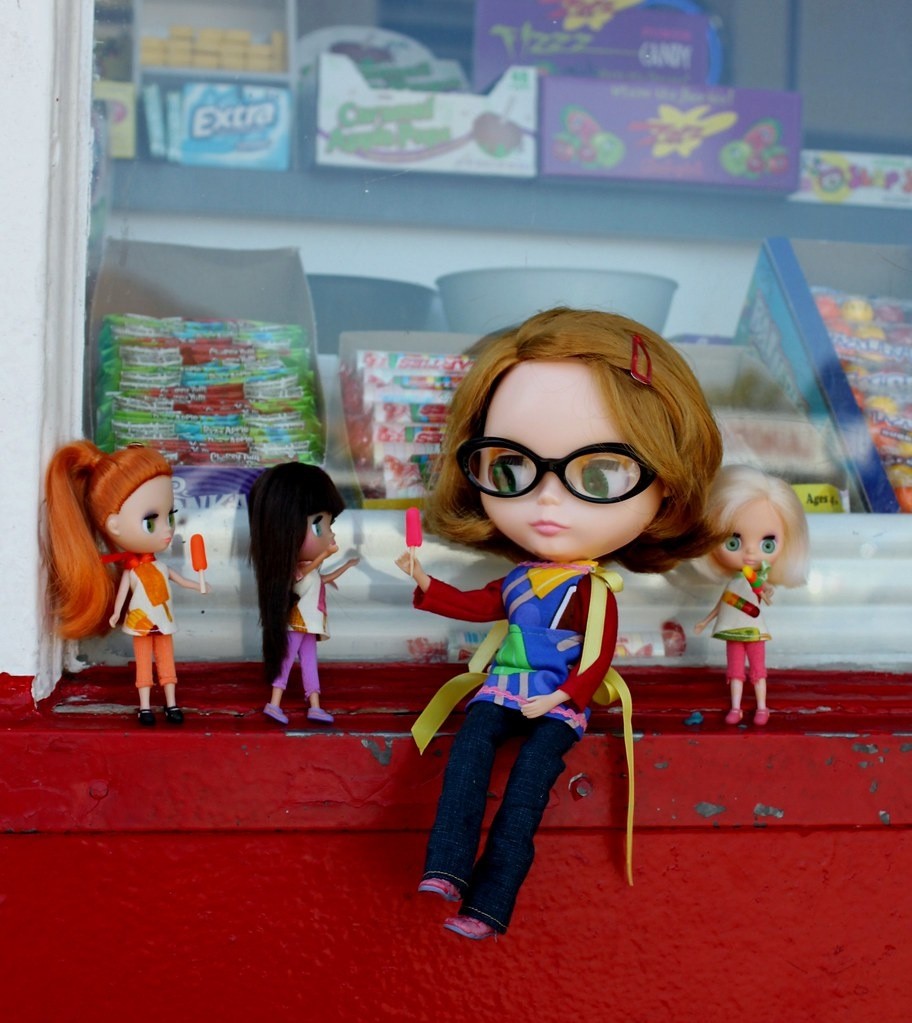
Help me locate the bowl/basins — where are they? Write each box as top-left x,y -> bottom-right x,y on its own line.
436,266 -> 681,344
303,273 -> 440,354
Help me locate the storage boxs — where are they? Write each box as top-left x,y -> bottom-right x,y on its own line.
309,50 -> 541,179
334,326 -> 482,512
179,81 -> 295,175
672,341 -> 851,512
790,146 -> 912,212
733,233 -> 912,518
83,236 -> 330,469
543,73 -> 807,197
88,0 -> 141,162
138,0 -> 300,80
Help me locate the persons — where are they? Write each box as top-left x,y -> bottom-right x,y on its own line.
246,461 -> 361,724
394,307 -> 722,939
694,465 -> 810,724
39,439 -> 210,724
